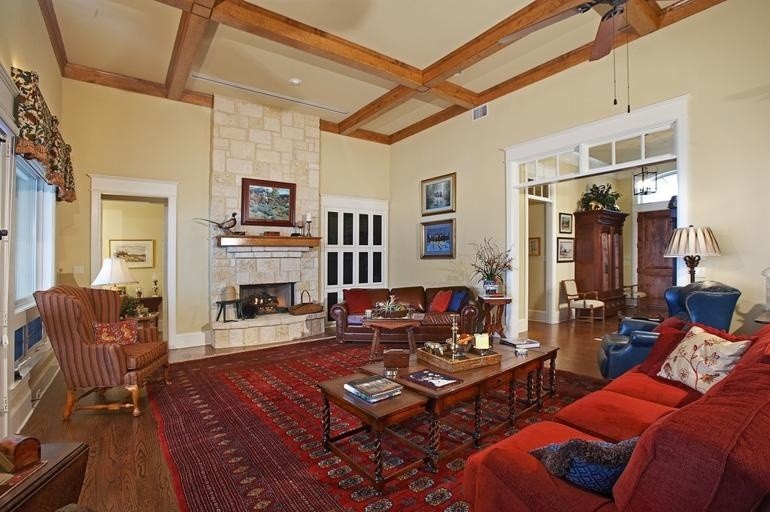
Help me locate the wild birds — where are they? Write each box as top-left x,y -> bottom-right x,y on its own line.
194,212 -> 237,234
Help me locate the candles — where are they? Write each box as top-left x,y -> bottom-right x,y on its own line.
306,213 -> 311,221
152,270 -> 157,281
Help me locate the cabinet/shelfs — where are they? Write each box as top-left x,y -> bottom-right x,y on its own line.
638,209 -> 677,311
573,211 -> 630,319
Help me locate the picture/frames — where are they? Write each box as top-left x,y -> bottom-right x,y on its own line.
241,178 -> 296,227
529,237 -> 540,256
557,213 -> 576,263
109,240 -> 154,268
420,172 -> 456,259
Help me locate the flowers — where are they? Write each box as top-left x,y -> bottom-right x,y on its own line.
468,236 -> 514,285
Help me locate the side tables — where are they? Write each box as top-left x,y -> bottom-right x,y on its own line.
478,294 -> 512,338
121,311 -> 161,343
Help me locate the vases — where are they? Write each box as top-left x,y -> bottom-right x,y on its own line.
483,280 -> 499,296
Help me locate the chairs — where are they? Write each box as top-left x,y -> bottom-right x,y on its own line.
623,284 -> 648,312
597,279 -> 742,381
561,280 -> 606,332
32,286 -> 173,419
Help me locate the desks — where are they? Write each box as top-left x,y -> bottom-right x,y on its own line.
754,312 -> 770,323
0,441 -> 90,512
133,297 -> 162,331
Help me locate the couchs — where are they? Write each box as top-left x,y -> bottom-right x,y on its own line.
330,286 -> 478,344
462,317 -> 770,512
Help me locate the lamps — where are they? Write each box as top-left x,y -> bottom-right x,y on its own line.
90,257 -> 139,297
633,165 -> 657,195
662,225 -> 723,284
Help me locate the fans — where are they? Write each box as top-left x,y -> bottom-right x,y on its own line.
496,0 -> 630,113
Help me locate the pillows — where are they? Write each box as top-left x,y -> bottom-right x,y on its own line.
90,318 -> 142,346
526,436 -> 639,500
649,317 -> 758,395
343,287 -> 468,314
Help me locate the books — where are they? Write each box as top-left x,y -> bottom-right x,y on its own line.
401,368 -> 464,393
343,373 -> 404,404
500,338 -> 541,348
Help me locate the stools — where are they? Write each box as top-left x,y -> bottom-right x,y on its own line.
216,299 -> 245,323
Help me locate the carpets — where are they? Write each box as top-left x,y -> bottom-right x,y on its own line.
146,337 -> 614,512
